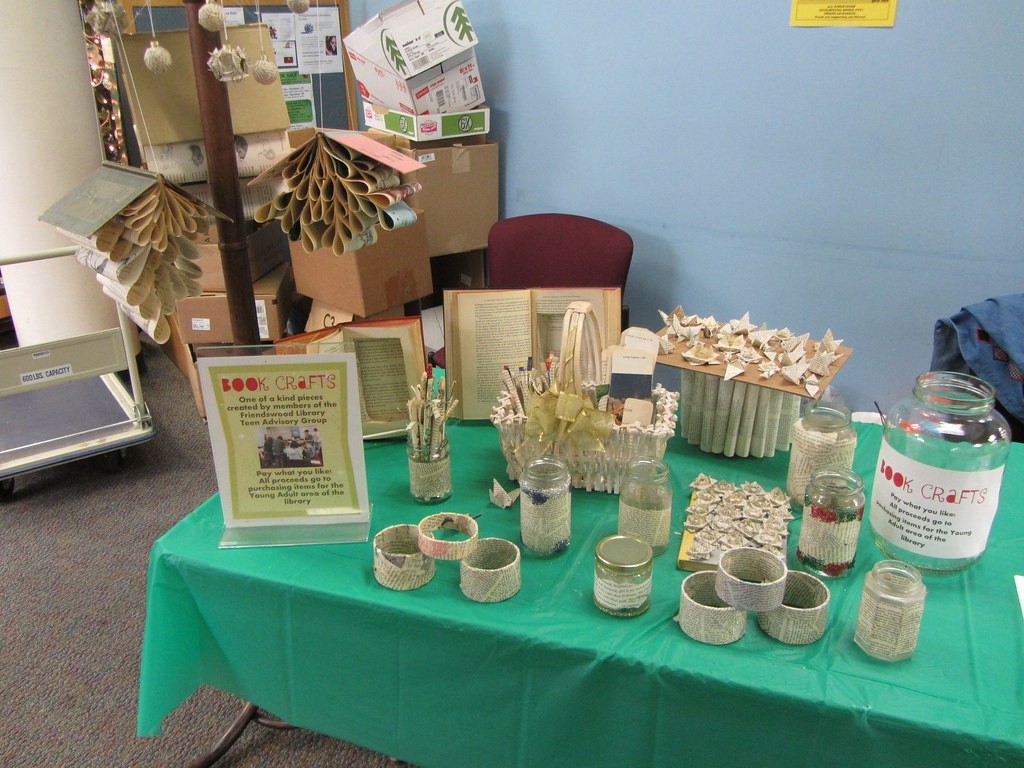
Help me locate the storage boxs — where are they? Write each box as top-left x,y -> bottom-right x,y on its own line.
112,0 -> 500,424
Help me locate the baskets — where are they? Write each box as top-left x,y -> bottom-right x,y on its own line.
490,300 -> 680,494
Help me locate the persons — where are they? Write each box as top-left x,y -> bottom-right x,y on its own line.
263,428 -> 320,468
326,36 -> 337,55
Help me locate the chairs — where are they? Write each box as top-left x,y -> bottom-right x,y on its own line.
488,213 -> 634,333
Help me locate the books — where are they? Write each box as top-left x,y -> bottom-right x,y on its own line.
444,286 -> 621,420
117,20 -> 292,224
247,132 -> 428,254
37,160 -> 237,343
273,315 -> 426,439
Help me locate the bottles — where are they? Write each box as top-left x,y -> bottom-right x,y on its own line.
593,535 -> 653,618
617,455 -> 672,556
407,434 -> 453,504
786,400 -> 858,508
519,454 -> 572,556
854,560 -> 926,661
796,465 -> 865,578
867,370 -> 1011,575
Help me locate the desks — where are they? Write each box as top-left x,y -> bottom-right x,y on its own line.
136,367 -> 1024,768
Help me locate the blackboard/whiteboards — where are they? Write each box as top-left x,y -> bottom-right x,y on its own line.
117,0 -> 358,130
